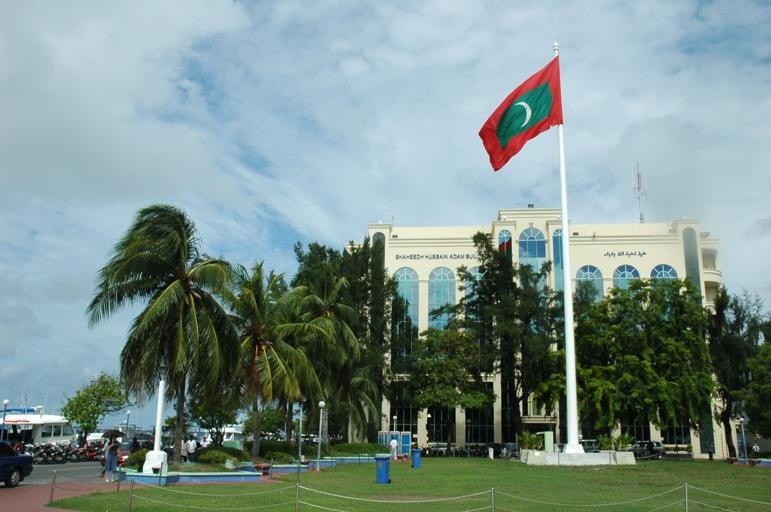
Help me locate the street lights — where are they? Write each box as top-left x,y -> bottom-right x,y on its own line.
1,399 -> 11,440
393,415 -> 399,431
740,418 -> 750,464
126,410 -> 131,440
316,400 -> 326,470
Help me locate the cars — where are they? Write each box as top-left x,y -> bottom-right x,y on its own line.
101,440 -> 143,467
264,429 -> 320,442
475,442 -> 519,458
0,441 -> 35,486
629,440 -> 663,459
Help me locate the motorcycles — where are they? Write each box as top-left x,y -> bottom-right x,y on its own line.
13,440 -> 107,464
419,445 -> 489,457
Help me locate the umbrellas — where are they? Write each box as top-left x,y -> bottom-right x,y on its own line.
101,429 -> 125,438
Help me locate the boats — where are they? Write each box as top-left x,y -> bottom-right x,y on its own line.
1,391 -> 105,445
205,428 -> 244,444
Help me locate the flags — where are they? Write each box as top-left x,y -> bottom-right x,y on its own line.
478,56 -> 563,172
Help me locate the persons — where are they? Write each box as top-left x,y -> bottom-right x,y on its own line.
101,441 -> 110,473
129,437 -> 141,453
180,432 -> 211,462
77,431 -> 84,447
103,435 -> 119,482
150,432 -> 155,444
389,437 -> 398,461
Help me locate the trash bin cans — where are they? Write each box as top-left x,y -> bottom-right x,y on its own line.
374,457 -> 391,484
411,448 -> 422,468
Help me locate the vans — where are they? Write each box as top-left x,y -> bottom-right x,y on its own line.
429,442 -> 456,452
579,439 -> 601,451
